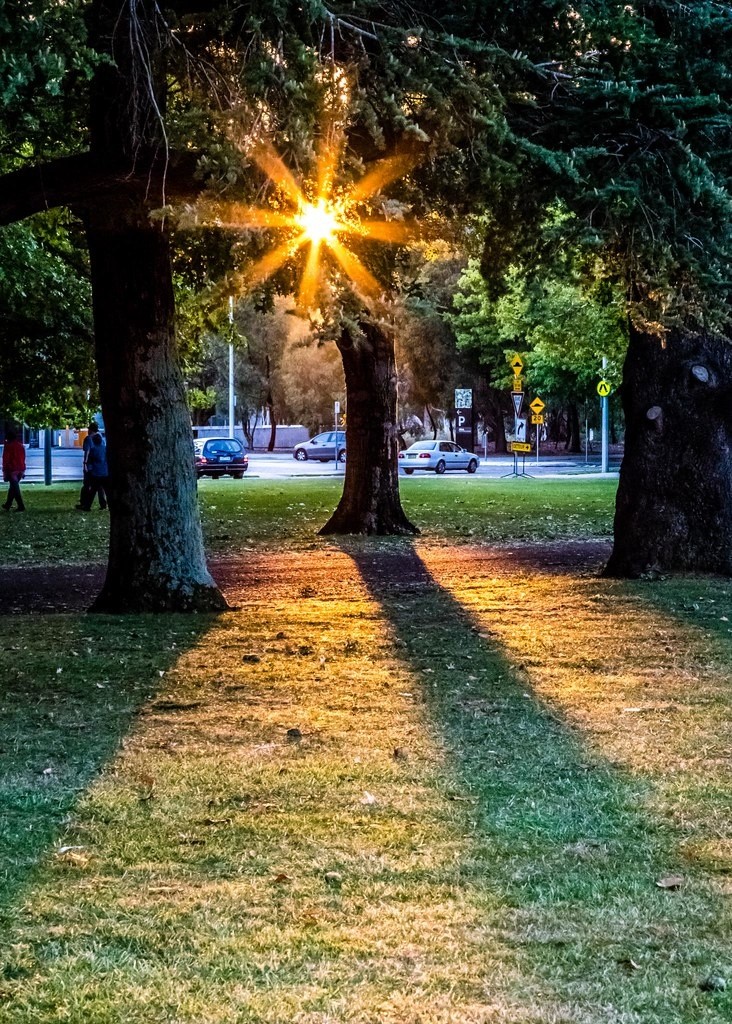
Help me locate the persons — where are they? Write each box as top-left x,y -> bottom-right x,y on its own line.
2,429 -> 26,511
80,434 -> 108,512
75,422 -> 107,510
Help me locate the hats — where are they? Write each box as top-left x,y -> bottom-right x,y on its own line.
87,424 -> 100,432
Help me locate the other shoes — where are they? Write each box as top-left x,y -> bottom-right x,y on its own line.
16,508 -> 25,511
76,505 -> 91,512
100,502 -> 106,509
2,504 -> 10,510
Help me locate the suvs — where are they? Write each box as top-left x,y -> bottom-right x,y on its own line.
193,437 -> 248,479
294,431 -> 347,462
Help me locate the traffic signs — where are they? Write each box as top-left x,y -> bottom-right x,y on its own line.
511,442 -> 531,452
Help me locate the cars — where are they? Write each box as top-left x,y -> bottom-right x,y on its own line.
397,440 -> 481,474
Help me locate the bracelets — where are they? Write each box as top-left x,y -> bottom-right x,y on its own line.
83,462 -> 87,464
